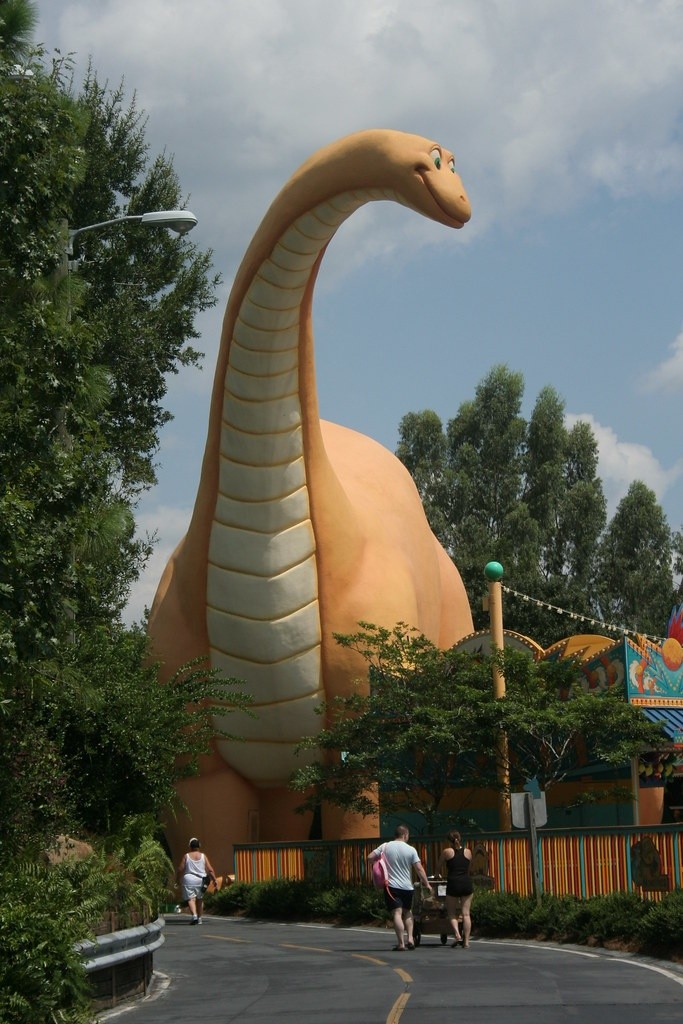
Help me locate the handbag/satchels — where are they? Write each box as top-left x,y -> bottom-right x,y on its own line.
201,876 -> 210,892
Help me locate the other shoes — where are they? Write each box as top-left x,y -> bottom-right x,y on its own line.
451,939 -> 463,948
408,943 -> 416,951
192,917 -> 197,923
463,944 -> 470,949
198,918 -> 202,925
393,946 -> 405,951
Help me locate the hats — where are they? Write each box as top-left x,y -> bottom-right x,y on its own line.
189,838 -> 199,848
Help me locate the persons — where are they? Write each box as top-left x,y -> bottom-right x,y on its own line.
173,838 -> 217,926
367,825 -> 433,951
434,830 -> 475,948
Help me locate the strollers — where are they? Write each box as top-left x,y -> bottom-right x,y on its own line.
411,876 -> 454,945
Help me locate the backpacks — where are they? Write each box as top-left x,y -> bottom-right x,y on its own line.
372,843 -> 399,904
